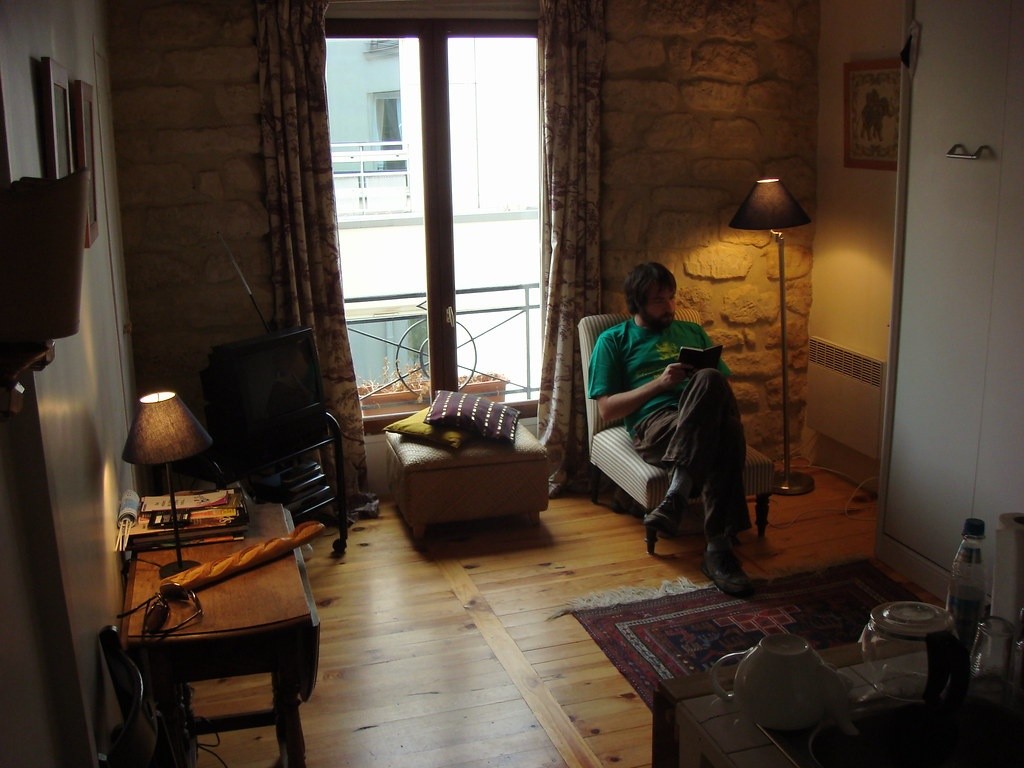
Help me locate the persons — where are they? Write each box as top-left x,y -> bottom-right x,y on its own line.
585,262 -> 756,599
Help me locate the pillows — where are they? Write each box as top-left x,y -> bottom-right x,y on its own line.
429,391 -> 520,444
384,407 -> 470,450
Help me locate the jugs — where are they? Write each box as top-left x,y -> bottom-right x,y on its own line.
859,601 -> 972,721
709,633 -> 860,738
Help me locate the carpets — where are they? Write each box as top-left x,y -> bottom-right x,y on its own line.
575,557 -> 923,712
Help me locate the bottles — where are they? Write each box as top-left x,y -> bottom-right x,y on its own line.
1009,639 -> 1024,696
969,617 -> 1012,692
946,518 -> 988,647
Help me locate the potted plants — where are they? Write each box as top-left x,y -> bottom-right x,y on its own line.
358,354 -> 510,414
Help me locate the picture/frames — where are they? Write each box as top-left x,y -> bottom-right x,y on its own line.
41,58 -> 100,251
844,57 -> 902,169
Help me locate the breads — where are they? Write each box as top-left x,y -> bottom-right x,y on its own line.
159,520 -> 326,590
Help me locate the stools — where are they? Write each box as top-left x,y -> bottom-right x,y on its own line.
385,419 -> 549,536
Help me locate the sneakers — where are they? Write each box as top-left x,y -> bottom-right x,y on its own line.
645,491 -> 687,531
701,545 -> 752,596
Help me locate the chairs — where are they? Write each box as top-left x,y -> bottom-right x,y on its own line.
576,288 -> 773,559
208,327 -> 351,551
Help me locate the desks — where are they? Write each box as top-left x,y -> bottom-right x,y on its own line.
653,641 -> 1024,768
122,489 -> 320,768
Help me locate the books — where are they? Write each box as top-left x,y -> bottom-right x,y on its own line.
117,486 -> 252,552
677,345 -> 724,369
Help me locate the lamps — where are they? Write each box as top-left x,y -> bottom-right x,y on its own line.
730,174 -> 818,495
121,392 -> 212,580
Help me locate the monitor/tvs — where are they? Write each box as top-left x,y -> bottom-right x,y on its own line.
200,324 -> 328,474
